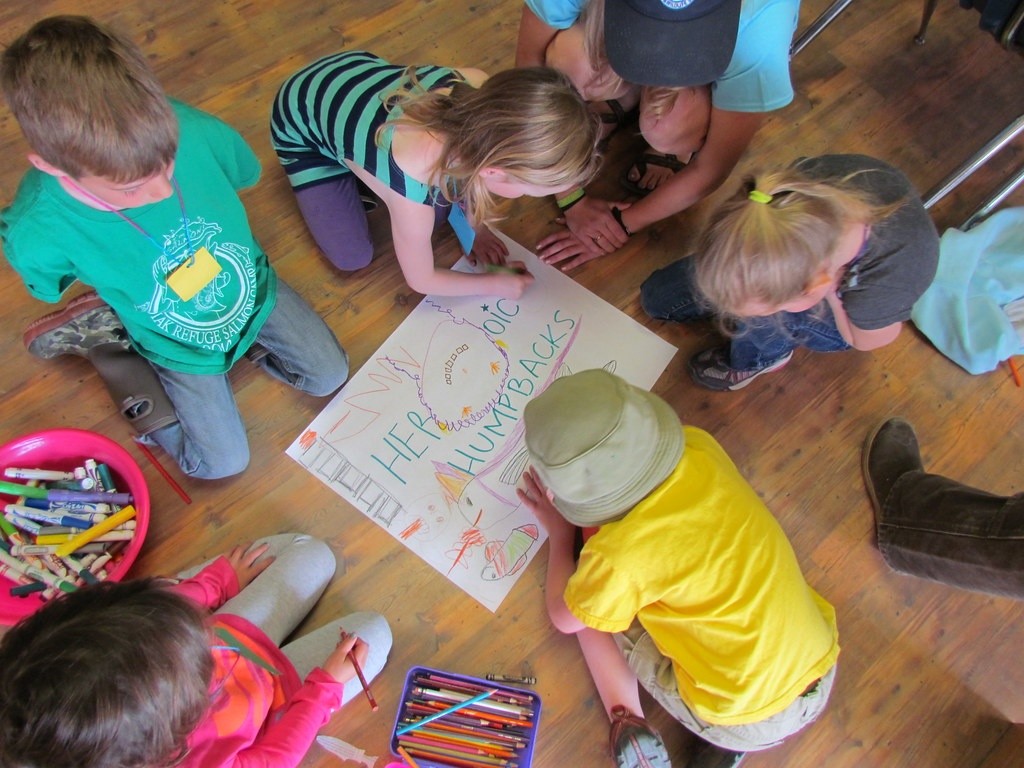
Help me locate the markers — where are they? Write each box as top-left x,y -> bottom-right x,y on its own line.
0,457 -> 139,603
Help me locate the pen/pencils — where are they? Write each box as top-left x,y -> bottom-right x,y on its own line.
1009,357 -> 1022,387
339,626 -> 379,712
128,432 -> 192,505
483,264 -> 526,274
394,670 -> 537,768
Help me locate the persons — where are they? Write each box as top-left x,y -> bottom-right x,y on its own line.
516,367 -> 842,767
269,50 -> 599,297
512,0 -> 801,272
640,152 -> 938,394
0,533 -> 392,767
1,15 -> 351,480
862,416 -> 1024,602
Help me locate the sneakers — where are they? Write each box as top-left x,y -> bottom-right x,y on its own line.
609,704 -> 671,768
695,739 -> 747,768
690,342 -> 793,390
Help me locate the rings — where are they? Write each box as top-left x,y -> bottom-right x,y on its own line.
596,235 -> 601,241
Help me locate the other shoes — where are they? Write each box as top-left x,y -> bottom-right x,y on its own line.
862,415 -> 921,536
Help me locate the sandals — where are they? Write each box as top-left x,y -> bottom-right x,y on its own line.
623,147 -> 695,194
594,98 -> 639,148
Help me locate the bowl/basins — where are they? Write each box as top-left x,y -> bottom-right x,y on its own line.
0,428 -> 148,629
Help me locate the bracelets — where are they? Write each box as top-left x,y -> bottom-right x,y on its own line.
557,187 -> 586,212
611,207 -> 633,237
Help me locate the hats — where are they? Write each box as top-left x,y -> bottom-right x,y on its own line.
525,368 -> 685,526
603,0 -> 741,86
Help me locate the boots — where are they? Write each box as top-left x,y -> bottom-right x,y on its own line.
24,291 -> 180,432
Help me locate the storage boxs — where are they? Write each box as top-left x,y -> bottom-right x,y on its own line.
386,666 -> 542,768
